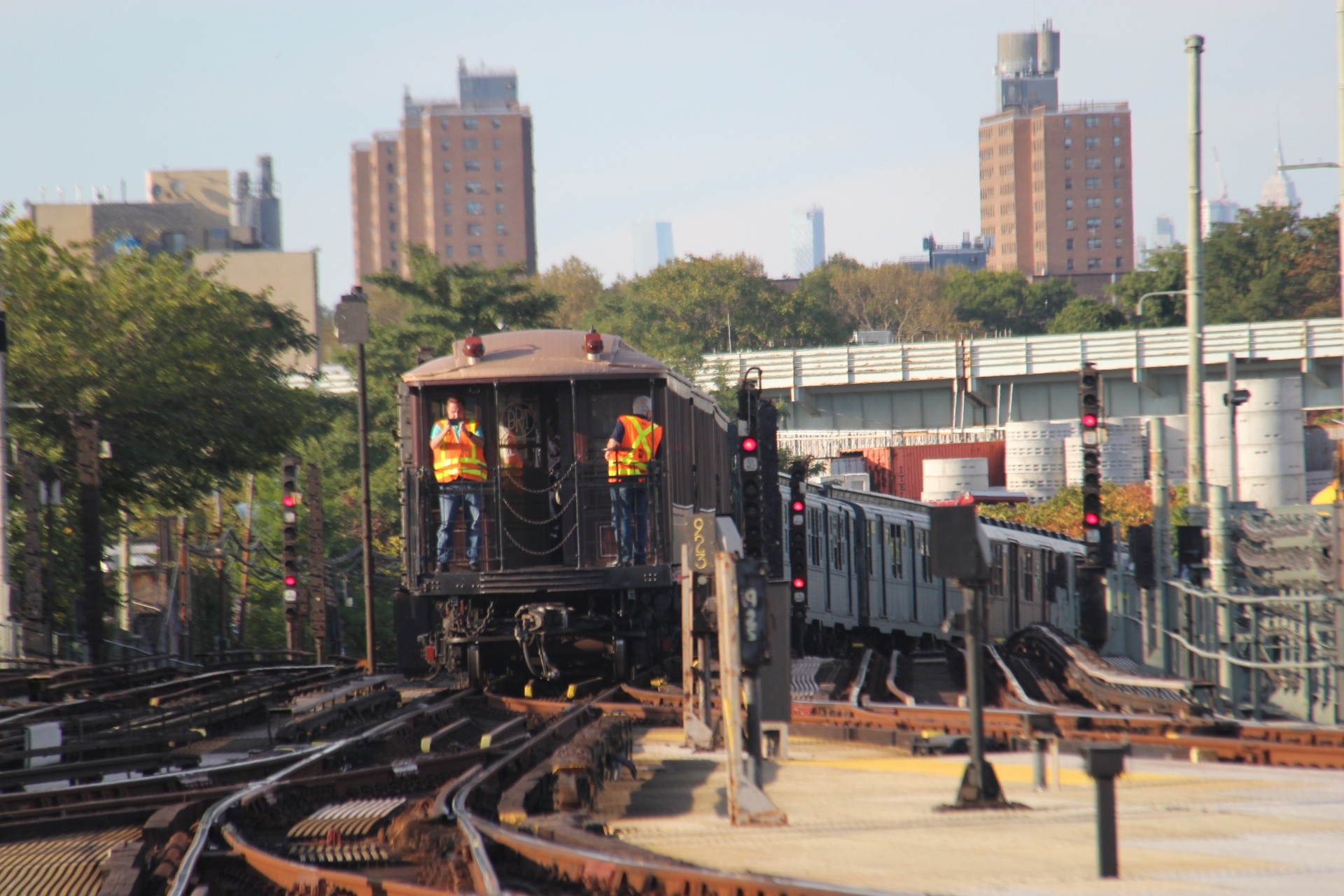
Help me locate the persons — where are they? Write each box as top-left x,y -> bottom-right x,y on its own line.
604,396 -> 663,568
429,397 -> 487,573
499,425 -> 523,469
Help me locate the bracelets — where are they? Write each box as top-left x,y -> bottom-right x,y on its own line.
468,432 -> 473,438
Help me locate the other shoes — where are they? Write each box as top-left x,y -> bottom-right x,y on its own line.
468,563 -> 480,572
611,559 -> 632,568
434,564 -> 449,574
631,560 -> 646,567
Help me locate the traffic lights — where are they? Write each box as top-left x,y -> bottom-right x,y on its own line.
279,455 -> 298,624
1078,362 -> 1102,569
787,458 -> 809,620
735,388 -> 764,562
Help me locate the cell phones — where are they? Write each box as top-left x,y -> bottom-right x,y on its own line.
449,418 -> 463,426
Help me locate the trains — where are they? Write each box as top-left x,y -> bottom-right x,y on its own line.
388,326 -> 1180,687
